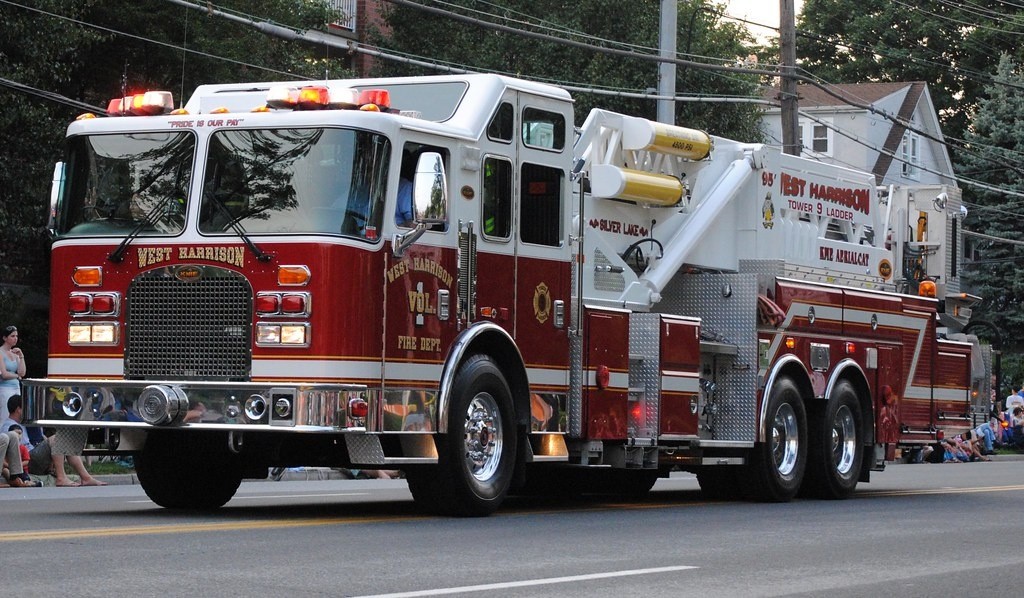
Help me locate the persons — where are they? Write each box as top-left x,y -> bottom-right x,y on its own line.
330,140 -> 417,241
0,424 -> 43,488
902,423 -> 1000,464
0,326 -> 26,434
990,375 -> 1024,454
0,394 -> 108,487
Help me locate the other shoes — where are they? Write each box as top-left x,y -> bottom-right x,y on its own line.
909,456 -> 991,464
984,440 -> 1019,454
8,474 -> 43,487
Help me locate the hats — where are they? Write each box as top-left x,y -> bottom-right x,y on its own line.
2,325 -> 17,338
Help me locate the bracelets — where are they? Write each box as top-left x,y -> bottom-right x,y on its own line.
19,356 -> 24,359
17,377 -> 19,379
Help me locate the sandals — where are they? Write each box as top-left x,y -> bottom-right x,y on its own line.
81,477 -> 107,486
56,479 -> 80,487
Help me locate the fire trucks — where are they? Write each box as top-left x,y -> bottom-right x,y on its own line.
21,69 -> 999,519
